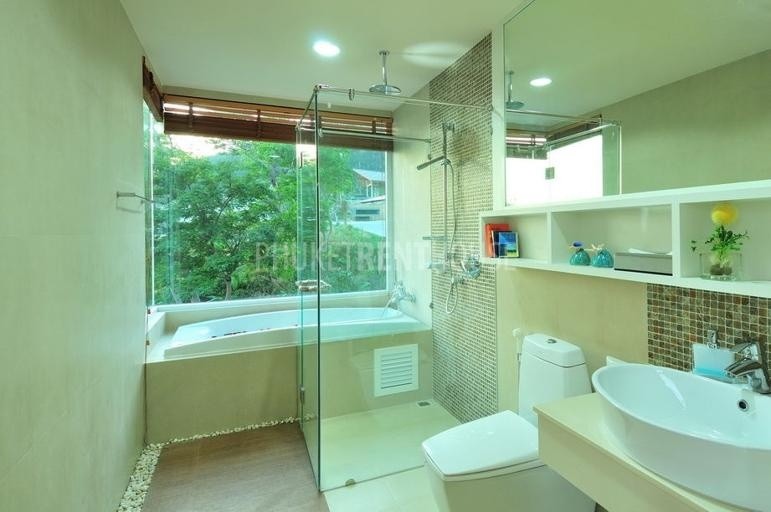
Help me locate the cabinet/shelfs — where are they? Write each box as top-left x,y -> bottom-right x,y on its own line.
478,179 -> 771,299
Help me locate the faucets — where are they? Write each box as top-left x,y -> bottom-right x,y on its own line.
725,342 -> 770,394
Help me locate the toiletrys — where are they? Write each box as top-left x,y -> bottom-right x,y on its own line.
692,329 -> 736,379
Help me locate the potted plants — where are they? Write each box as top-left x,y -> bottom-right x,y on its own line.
689,225 -> 753,281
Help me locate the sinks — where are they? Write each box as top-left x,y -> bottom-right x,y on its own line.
590,362 -> 771,512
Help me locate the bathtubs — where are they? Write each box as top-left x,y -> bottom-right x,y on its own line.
166,307 -> 424,449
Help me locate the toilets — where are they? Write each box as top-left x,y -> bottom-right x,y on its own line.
421,332 -> 597,512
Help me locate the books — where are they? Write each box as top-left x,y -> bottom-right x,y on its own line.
497,231 -> 520,260
484,222 -> 510,258
490,228 -> 512,259
